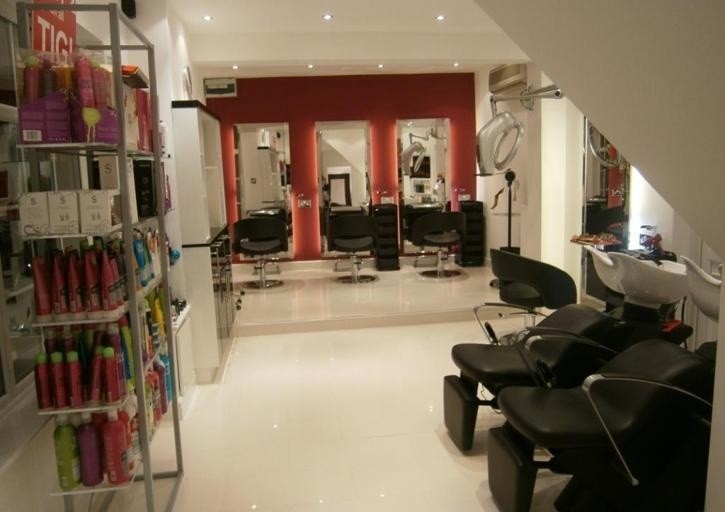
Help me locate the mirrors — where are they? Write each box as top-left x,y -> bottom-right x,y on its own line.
580,112 -> 631,311
234,119 -> 448,255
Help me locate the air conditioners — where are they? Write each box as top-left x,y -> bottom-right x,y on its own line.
487,63 -> 526,96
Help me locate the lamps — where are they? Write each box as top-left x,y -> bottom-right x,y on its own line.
475,86 -> 562,176
231,212 -> 467,290
587,122 -> 628,174
246,203 -> 444,251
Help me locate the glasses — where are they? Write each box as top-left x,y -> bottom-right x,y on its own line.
475,86 -> 562,176
587,122 -> 628,174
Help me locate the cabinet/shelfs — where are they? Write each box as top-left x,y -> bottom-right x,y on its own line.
35,325 -> 126,408
34,282 -> 165,413
22,146 -> 162,234
26,234 -> 165,321
18,3 -> 156,147
25,55 -> 56,107
455,200 -> 485,265
62,407 -> 181,511
44,344 -> 172,495
257,146 -> 287,204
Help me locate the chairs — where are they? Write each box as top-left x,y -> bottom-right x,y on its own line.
487,340 -> 712,512
231,212 -> 467,290
442,302 -> 629,450
474,248 -> 578,346
246,203 -> 444,251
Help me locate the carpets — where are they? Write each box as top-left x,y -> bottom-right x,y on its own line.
231,268 -> 531,336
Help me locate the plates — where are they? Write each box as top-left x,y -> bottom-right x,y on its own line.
257,146 -> 287,204
455,200 -> 485,265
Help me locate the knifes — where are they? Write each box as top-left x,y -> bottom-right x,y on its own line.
487,63 -> 526,96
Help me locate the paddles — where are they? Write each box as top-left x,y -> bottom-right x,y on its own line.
231,268 -> 531,336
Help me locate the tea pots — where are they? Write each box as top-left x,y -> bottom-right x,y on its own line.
580,112 -> 631,311
234,119 -> 448,255
